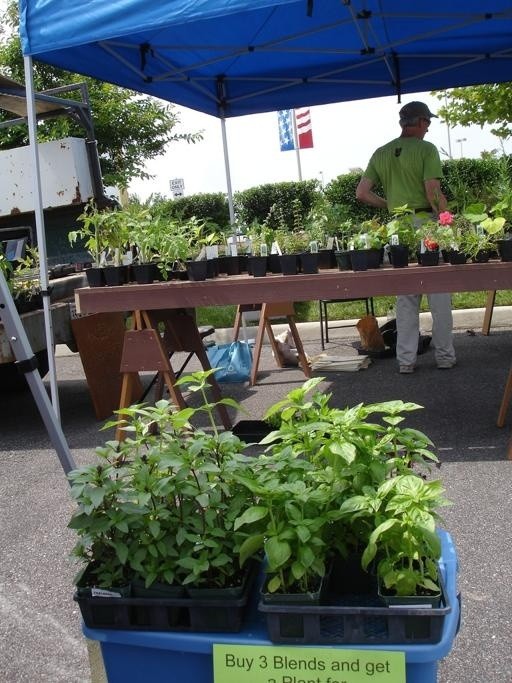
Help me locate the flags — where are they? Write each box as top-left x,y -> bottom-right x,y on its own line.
276,108 -> 295,151
295,106 -> 313,148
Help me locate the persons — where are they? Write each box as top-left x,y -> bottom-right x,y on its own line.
356,99 -> 457,374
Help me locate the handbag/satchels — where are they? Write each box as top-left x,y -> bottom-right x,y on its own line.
208,342 -> 251,384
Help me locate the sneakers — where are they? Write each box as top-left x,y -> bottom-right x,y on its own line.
399,364 -> 416,374
436,359 -> 453,368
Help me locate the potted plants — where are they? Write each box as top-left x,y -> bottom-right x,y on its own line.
2,246 -> 44,314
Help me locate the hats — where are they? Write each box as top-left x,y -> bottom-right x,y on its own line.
400,102 -> 439,118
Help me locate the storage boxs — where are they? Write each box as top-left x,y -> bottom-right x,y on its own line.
79,528 -> 463,681
